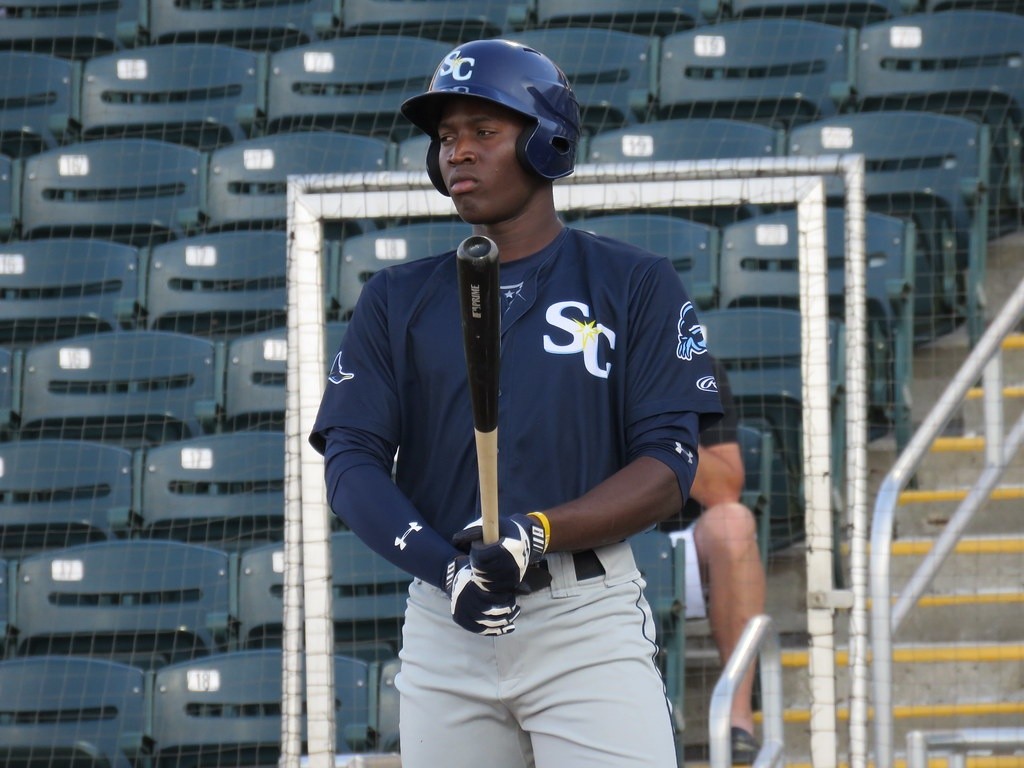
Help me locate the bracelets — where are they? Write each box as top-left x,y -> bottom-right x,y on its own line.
526,510 -> 551,557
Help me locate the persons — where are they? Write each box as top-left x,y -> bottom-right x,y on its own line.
649,350 -> 771,767
302,33 -> 736,765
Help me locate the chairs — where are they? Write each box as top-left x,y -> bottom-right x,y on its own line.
2,1 -> 1024,768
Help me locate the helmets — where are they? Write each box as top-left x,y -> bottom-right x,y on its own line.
400,38 -> 583,197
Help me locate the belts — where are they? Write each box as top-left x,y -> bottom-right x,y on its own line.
516,549 -> 605,595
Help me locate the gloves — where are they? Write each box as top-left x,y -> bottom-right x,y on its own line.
443,554 -> 521,636
451,512 -> 545,592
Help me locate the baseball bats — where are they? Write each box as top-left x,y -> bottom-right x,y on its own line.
457,235 -> 501,544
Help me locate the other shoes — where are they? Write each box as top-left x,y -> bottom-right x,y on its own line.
731,726 -> 757,764
